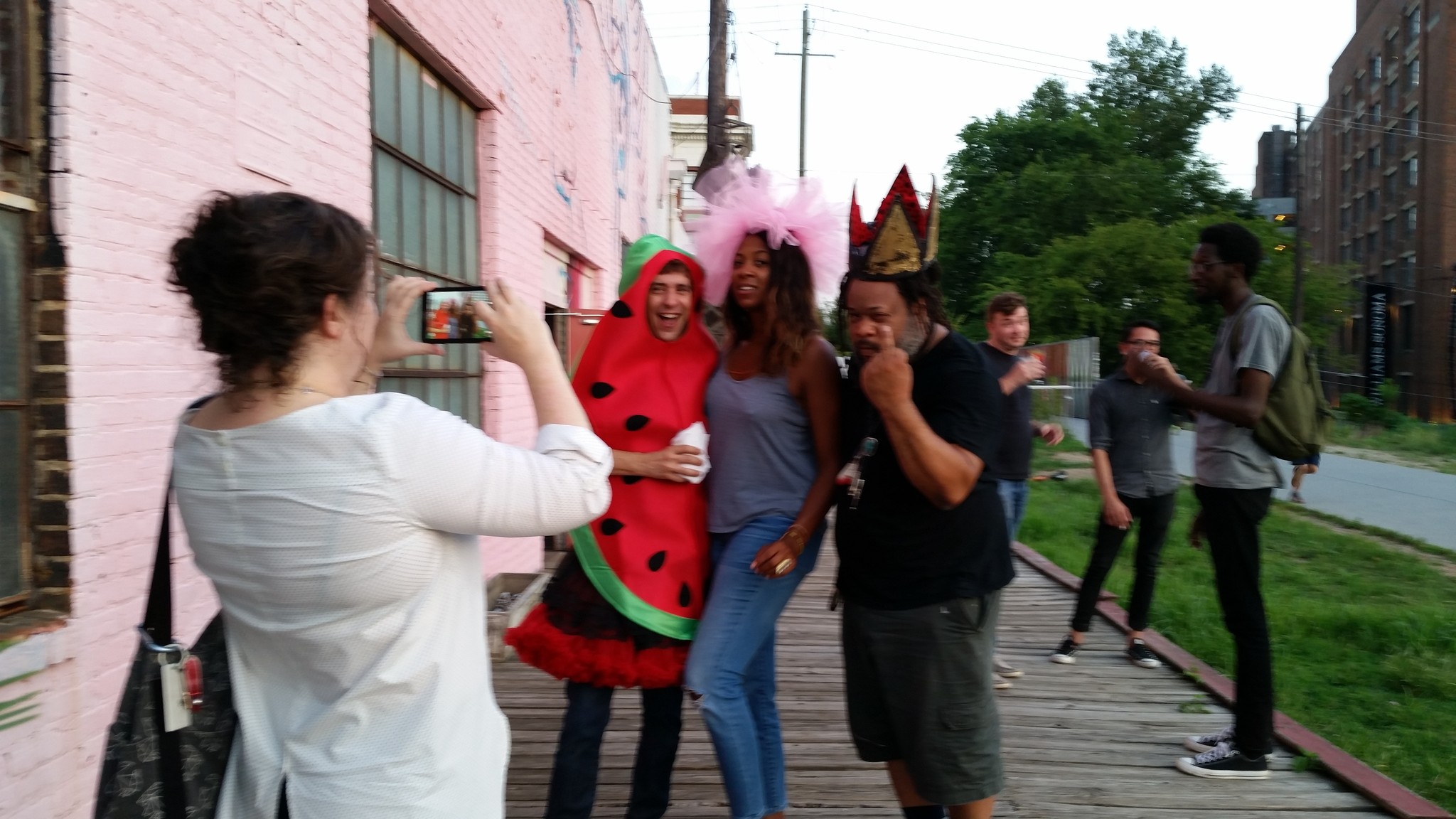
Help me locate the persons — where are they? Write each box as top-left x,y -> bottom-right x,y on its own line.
1289,351 -> 1339,502
1145,223 -> 1290,781
1052,321 -> 1190,669
505,234 -> 724,819
687,157 -> 844,819
835,163 -> 1033,819
423,298 -> 482,340
976,294 -> 1065,687
168,193 -> 614,819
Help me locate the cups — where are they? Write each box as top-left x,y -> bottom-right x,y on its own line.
1138,350 -> 1151,362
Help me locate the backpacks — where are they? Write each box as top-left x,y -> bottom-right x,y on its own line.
1228,297 -> 1338,460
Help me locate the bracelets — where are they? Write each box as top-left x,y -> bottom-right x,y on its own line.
780,524 -> 808,556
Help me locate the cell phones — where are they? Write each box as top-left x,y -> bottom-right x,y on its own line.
421,286 -> 497,345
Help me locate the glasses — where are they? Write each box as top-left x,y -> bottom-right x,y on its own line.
1188,263 -> 1221,274
1128,337 -> 1161,351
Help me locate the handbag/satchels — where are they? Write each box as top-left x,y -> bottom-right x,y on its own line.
95,609 -> 237,819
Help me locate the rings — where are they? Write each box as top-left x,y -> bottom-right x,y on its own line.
776,559 -> 794,575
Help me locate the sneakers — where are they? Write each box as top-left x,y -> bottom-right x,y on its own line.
1186,727 -> 1276,762
1175,742 -> 1269,782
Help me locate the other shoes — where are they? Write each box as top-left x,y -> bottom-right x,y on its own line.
992,658 -> 1023,677
1292,465 -> 1302,473
1053,634 -> 1084,663
1123,637 -> 1162,667
991,672 -> 1015,689
1291,491 -> 1307,504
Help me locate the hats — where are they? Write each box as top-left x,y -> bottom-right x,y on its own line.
847,164 -> 939,277
